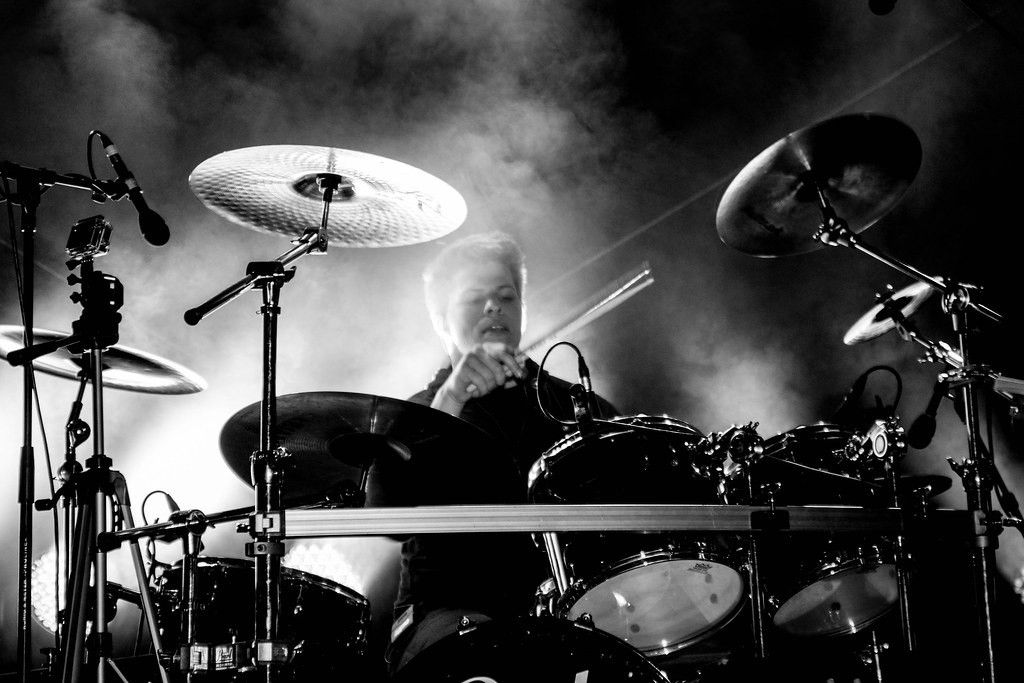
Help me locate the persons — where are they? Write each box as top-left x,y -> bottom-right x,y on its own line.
364,230 -> 624,678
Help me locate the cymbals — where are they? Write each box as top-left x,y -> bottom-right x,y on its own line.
188,142 -> 468,250
0,324 -> 209,396
842,275 -> 944,346
220,390 -> 521,508
713,112 -> 922,259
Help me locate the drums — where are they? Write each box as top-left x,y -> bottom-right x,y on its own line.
146,557 -> 371,683
389,615 -> 670,683
524,411 -> 751,657
726,421 -> 898,639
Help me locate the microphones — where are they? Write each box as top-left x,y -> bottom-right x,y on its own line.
907,381 -> 950,450
577,357 -> 602,420
840,372 -> 868,411
99,135 -> 171,246
166,493 -> 206,555
